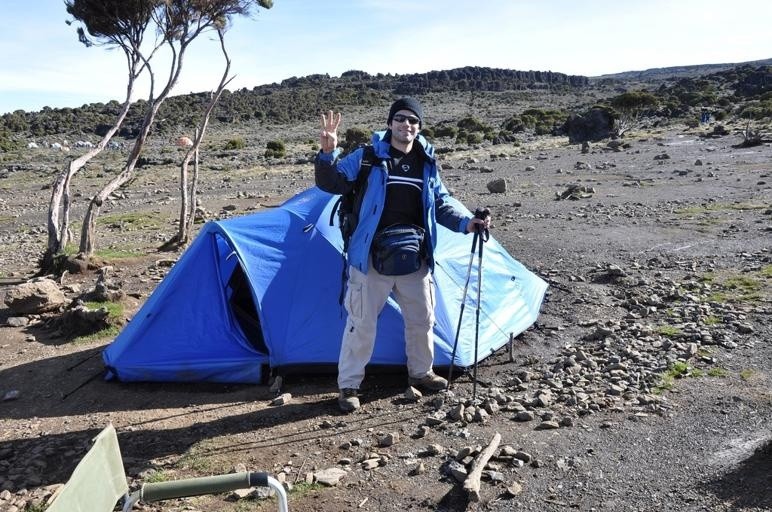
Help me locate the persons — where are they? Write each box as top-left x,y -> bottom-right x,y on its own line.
314,96 -> 491,412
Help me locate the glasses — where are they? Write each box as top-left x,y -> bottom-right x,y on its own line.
392,114 -> 420,124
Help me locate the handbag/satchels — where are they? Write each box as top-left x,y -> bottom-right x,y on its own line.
372,223 -> 426,276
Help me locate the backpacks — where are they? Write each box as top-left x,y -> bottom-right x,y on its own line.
329,143 -> 376,253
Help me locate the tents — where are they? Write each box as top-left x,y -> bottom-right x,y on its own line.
99,182 -> 551,387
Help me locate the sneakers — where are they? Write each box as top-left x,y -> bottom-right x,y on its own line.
408,372 -> 448,390
337,389 -> 360,410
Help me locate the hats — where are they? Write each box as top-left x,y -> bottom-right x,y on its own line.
387,98 -> 423,130
